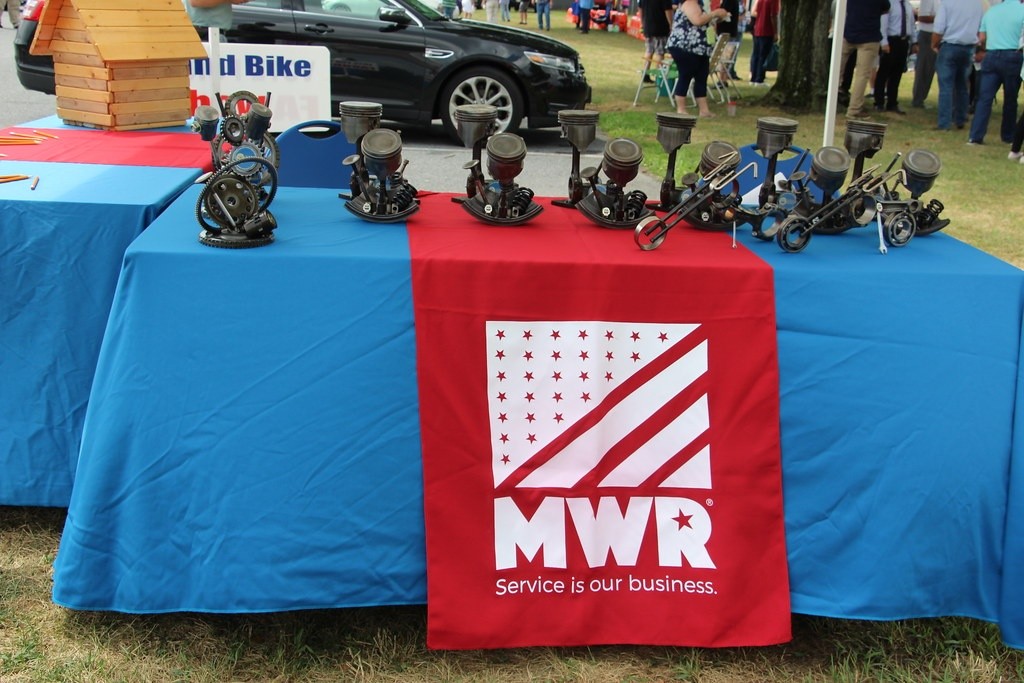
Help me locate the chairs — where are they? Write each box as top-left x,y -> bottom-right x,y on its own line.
262,121 -> 358,189
633,33 -> 742,109
698,144 -> 840,204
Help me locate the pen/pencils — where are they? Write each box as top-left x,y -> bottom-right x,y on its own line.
0,175 -> 30,183
10,132 -> 47,140
0,136 -> 43,145
33,130 -> 59,139
31,176 -> 39,190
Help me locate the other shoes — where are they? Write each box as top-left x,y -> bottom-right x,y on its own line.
886,104 -> 906,115
750,81 -> 765,86
643,74 -> 655,84
1008,151 -> 1024,163
711,81 -> 729,90
1002,142 -> 1012,146
846,112 -> 870,120
967,139 -> 983,145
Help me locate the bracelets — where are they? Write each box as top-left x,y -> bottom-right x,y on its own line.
981,48 -> 986,51
711,11 -> 718,18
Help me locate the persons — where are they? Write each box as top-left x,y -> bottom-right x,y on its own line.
0,0 -> 21,28
437,0 -> 630,34
638,0 -> 1024,164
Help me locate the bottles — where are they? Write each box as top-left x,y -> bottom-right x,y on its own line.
728,102 -> 737,115
706,25 -> 715,44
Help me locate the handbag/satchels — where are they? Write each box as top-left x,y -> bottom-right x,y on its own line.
763,44 -> 780,70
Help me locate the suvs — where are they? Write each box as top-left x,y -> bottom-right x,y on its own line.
11,0 -> 594,145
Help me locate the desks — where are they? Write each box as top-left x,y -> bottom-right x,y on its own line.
1,113 -> 246,507
52,181 -> 1023,655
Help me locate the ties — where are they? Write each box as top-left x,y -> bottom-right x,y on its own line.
900,0 -> 907,37
